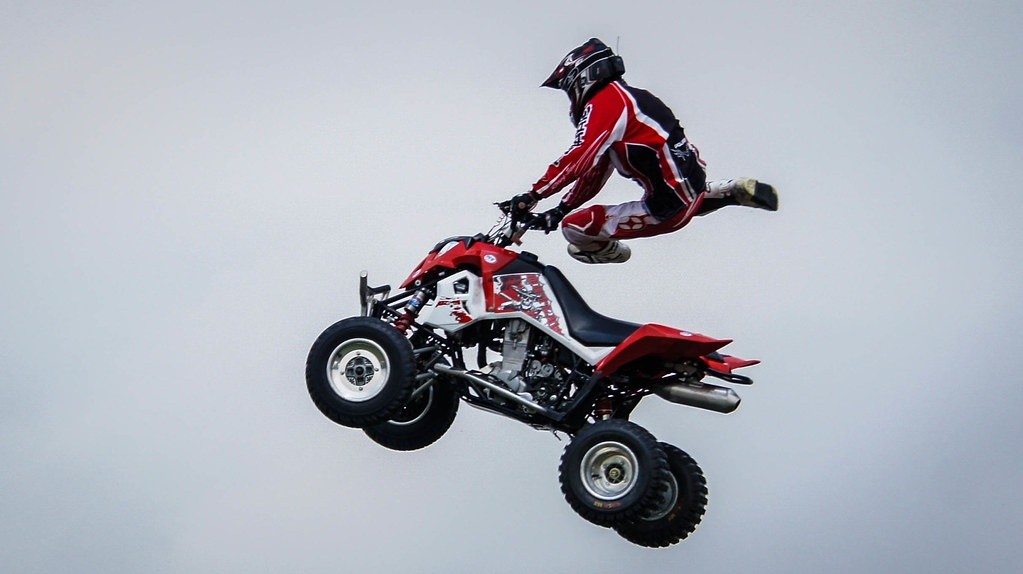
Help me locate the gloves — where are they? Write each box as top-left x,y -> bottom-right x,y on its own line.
498,189 -> 542,229
529,200 -> 573,234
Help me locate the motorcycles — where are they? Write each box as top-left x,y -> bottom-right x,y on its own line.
306,199 -> 761,549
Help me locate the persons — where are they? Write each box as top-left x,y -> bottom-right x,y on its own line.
499,38 -> 779,264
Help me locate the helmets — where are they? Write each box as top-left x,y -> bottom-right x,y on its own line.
539,38 -> 626,128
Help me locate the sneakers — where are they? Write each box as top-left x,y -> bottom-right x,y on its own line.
567,241 -> 631,265
720,176 -> 779,212
